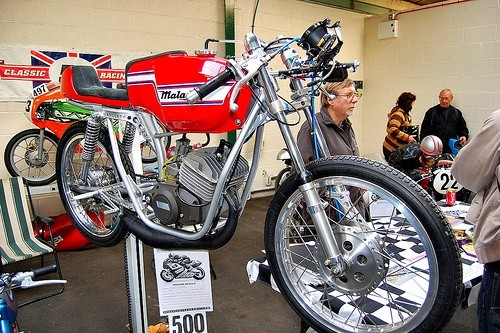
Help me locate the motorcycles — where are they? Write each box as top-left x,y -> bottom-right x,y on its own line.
160,257 -> 206,282
405,153 -> 459,200
3,79 -> 173,188
54,17 -> 465,333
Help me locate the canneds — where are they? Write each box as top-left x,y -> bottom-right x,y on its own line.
446,189 -> 456,206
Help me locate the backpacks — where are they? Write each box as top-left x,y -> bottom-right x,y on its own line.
388,143 -> 426,171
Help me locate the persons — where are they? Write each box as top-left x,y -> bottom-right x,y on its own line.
383,92 -> 416,161
419,88 -> 470,154
450,108 -> 500,332
291,75 -> 370,243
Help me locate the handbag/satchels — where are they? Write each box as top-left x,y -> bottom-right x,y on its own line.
397,126 -> 419,143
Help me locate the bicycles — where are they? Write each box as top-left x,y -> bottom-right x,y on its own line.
0,264 -> 67,333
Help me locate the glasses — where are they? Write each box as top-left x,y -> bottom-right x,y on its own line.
337,93 -> 362,100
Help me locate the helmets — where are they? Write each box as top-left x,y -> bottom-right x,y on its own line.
420,135 -> 443,156
